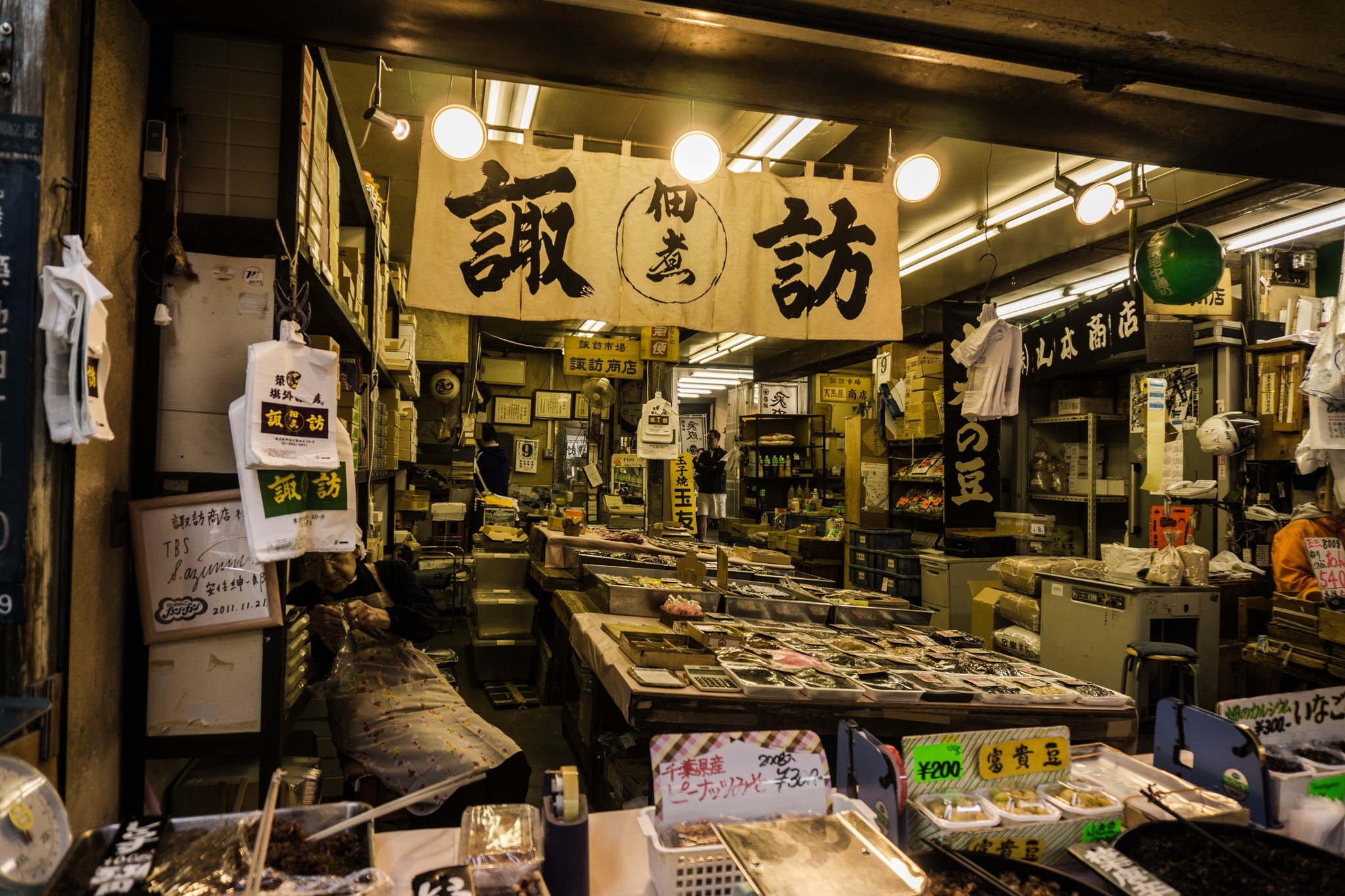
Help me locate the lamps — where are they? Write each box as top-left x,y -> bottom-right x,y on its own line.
363,106 -> 410,141
1054,174 -> 1118,225
670,130 -> 722,183
1111,192 -> 1155,215
431,103 -> 488,162
888,151 -> 941,203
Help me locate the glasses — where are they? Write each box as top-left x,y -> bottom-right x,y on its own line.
304,551 -> 349,572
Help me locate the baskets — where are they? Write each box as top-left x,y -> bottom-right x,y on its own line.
636,793 -> 881,896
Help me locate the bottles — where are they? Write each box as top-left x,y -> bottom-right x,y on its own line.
803,524 -> 807,532
757,456 -> 763,477
772,455 -> 778,476
812,488 -> 819,500
787,486 -> 795,510
790,497 -> 800,513
778,456 -> 785,477
785,455 -> 791,477
798,524 -> 804,533
796,485 -> 804,508
806,492 -> 816,512
614,469 -> 643,497
804,486 -> 810,509
551,502 -> 554,510
743,457 -> 748,476
807,524 -> 811,531
792,453 -> 799,477
764,455 -> 770,476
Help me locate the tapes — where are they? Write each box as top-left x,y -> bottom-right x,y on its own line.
561,766 -> 580,821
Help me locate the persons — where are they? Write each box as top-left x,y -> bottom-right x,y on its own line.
693,448 -> 704,474
695,429 -> 727,543
1270,471 -> 1344,592
287,522 -> 534,827
473,422 -> 511,534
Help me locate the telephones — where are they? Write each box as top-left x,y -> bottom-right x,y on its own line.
1166,480 -> 1216,496
1244,506 -> 1292,521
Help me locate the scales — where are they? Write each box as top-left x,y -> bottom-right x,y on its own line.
0,697 -> 73,896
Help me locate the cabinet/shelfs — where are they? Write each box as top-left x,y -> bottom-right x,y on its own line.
115,17 -> 409,851
1028,413 -> 1130,560
738,413 -> 827,524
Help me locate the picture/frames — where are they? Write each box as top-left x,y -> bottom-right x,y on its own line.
492,394 -> 534,427
532,388 -> 575,421
480,356 -> 527,386
572,390 -> 612,422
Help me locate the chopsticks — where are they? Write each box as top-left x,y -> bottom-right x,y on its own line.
918,837 -> 1023,896
243,768 -> 283,896
305,765 -> 488,841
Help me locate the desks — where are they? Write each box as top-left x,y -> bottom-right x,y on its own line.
529,520 -> 1139,814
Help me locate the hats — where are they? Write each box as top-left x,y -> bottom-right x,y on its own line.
355,522 -> 364,546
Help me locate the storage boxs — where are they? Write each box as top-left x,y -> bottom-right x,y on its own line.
394,489 -> 431,511
896,353 -> 944,439
1058,397 -> 1114,415
465,532 -> 539,681
167,31 -> 422,473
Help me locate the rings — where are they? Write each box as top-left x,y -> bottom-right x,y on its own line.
355,617 -> 358,625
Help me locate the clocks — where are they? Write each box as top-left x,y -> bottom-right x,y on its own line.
618,372 -> 668,434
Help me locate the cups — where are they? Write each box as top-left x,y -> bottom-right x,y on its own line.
531,508 -> 540,515
549,509 -> 555,517
542,509 -> 549,516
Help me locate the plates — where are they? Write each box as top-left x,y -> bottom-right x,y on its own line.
907,849 -> 1112,896
1111,820 -> 1344,896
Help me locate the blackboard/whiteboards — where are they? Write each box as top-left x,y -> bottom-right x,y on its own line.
129,486 -> 284,646
